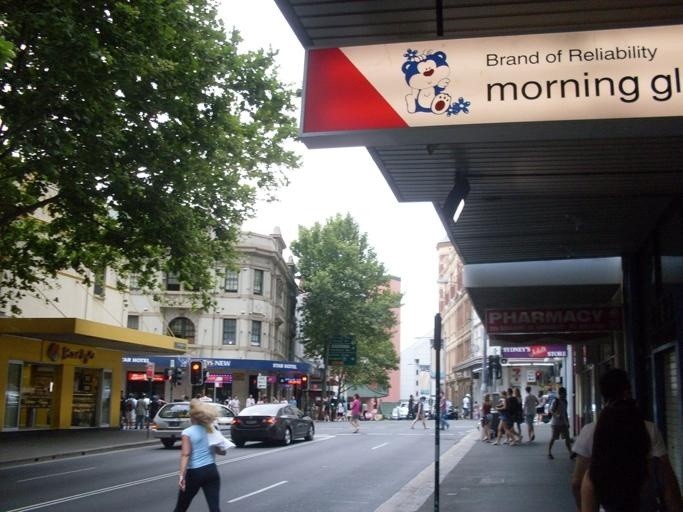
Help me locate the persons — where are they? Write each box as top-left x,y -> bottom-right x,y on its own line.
406,384 -> 575,459
572,368 -> 673,512
171,397 -> 221,511
580,402 -> 682,511
120,389 -> 376,434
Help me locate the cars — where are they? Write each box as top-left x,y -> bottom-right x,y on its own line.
232,403 -> 316,448
391,401 -> 430,420
430,400 -> 458,419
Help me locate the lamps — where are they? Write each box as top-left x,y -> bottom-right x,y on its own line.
443,180 -> 471,224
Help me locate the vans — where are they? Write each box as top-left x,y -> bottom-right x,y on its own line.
152,403 -> 238,448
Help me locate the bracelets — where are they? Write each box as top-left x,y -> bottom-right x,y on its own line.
179,475 -> 185,477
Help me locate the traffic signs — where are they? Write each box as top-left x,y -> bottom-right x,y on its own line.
328,335 -> 356,365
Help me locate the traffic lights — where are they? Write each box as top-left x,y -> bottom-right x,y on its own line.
174,369 -> 182,386
190,361 -> 203,384
302,373 -> 309,391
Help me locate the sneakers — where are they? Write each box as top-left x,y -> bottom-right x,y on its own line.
482,435 -> 536,446
549,454 -> 554,459
570,451 -> 577,459
